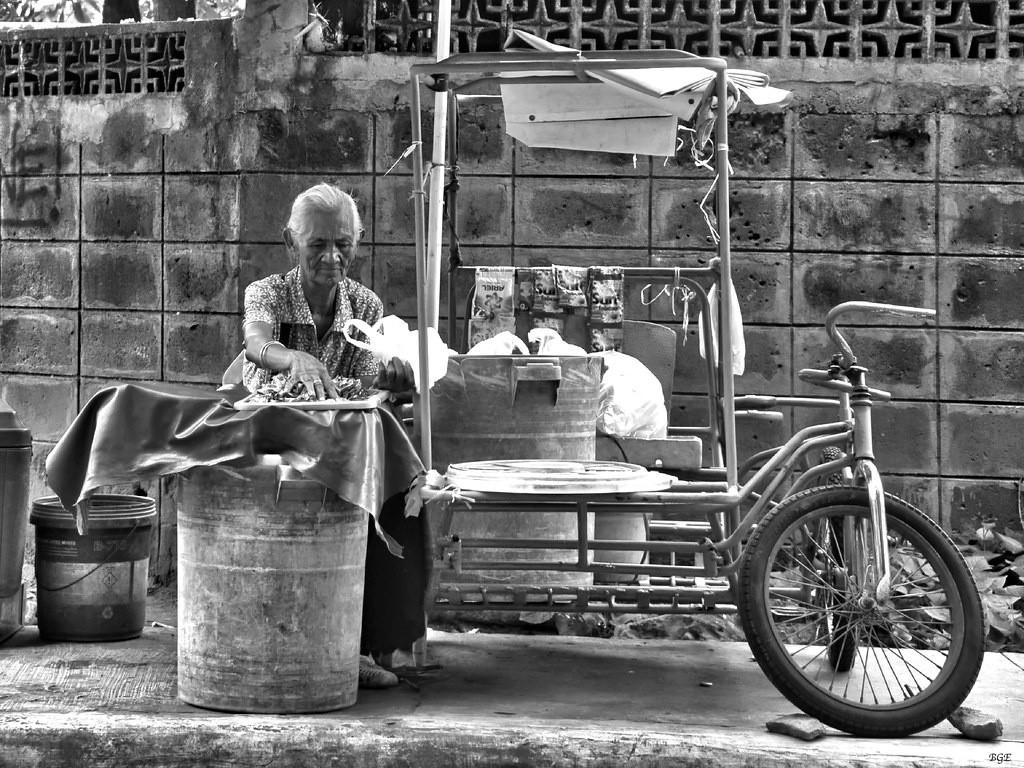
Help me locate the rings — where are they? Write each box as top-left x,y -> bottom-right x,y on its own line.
313,380 -> 322,383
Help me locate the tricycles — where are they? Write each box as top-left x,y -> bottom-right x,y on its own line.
389,48 -> 990,742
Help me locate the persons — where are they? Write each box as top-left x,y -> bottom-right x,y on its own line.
241,181 -> 416,691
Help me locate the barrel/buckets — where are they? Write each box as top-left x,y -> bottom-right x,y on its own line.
176,454 -> 368,711
410,355 -> 602,602
29,493 -> 156,638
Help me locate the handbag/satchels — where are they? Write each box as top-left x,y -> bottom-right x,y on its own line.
468,327 -> 668,441
343,313 -> 449,396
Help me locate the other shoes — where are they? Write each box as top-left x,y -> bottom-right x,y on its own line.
359,654 -> 398,688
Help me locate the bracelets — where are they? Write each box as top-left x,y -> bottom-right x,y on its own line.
259,340 -> 286,373
